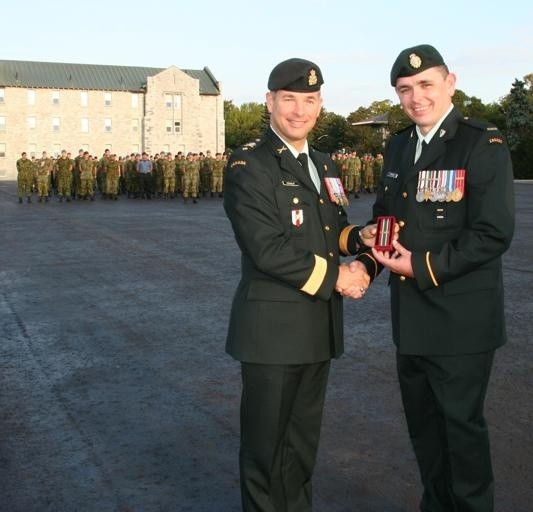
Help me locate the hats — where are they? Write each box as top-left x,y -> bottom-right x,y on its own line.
390,44 -> 445,88
267,57 -> 324,93
31,150 -> 227,163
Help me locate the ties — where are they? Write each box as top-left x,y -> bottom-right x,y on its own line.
298,153 -> 313,182
421,139 -> 428,154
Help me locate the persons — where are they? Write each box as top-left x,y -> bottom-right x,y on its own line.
333,44 -> 516,512
222,58 -> 406,512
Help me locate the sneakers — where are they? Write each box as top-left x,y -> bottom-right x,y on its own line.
26,196 -> 33,203
183,197 -> 198,205
354,187 -> 377,199
37,197 -> 48,202
17,196 -> 22,204
57,193 -> 223,202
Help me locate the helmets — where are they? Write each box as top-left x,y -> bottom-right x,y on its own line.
332,148 -> 373,156
